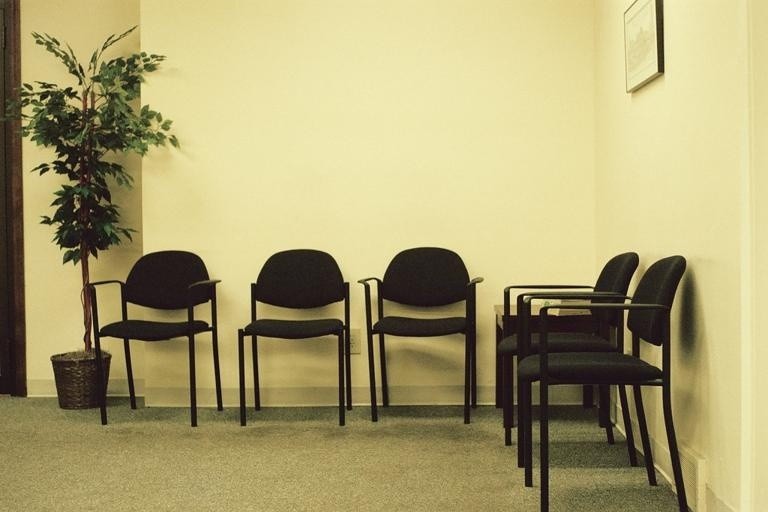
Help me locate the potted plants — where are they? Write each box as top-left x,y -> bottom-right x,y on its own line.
1,25 -> 181,410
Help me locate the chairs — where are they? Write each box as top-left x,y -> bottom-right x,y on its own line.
238,249 -> 351,426
89,250 -> 222,426
358,246 -> 484,424
498,252 -> 639,467
524,255 -> 688,512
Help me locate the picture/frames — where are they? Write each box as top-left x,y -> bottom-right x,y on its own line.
623,0 -> 664,93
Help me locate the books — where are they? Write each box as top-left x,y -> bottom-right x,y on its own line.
547,299 -> 592,317
